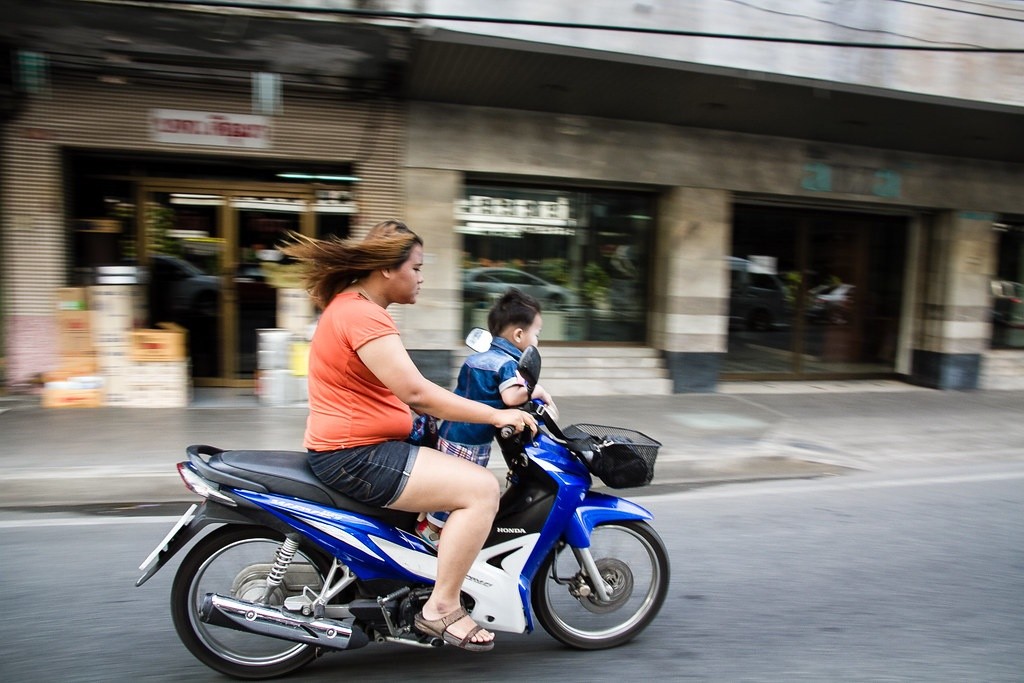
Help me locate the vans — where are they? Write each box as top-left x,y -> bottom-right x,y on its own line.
720,253 -> 795,330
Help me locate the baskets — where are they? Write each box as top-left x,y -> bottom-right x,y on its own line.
566,424 -> 659,489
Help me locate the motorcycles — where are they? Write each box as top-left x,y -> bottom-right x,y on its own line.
127,325 -> 672,679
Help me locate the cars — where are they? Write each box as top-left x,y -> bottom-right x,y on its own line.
465,264 -> 578,310
123,249 -> 239,322
809,278 -> 859,317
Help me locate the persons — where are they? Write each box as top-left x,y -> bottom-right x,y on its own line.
414,285 -> 559,555
271,218 -> 503,654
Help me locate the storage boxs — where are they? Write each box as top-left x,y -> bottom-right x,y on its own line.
37,282 -> 325,408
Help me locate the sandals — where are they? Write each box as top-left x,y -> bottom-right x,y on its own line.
415,607 -> 494,651
